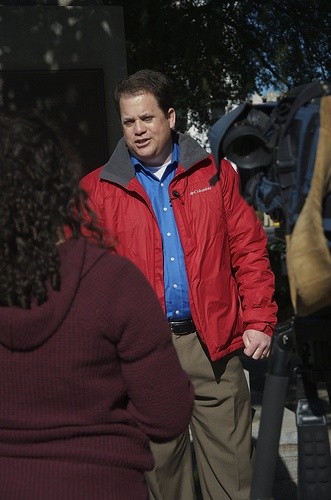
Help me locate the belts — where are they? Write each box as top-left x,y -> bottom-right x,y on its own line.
168,320 -> 196,335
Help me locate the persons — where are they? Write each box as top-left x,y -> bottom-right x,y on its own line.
65,69 -> 278,500
0,116 -> 193,500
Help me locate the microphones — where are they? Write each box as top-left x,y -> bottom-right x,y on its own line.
172,190 -> 181,197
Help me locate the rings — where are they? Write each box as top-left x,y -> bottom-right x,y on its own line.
262,353 -> 267,357
